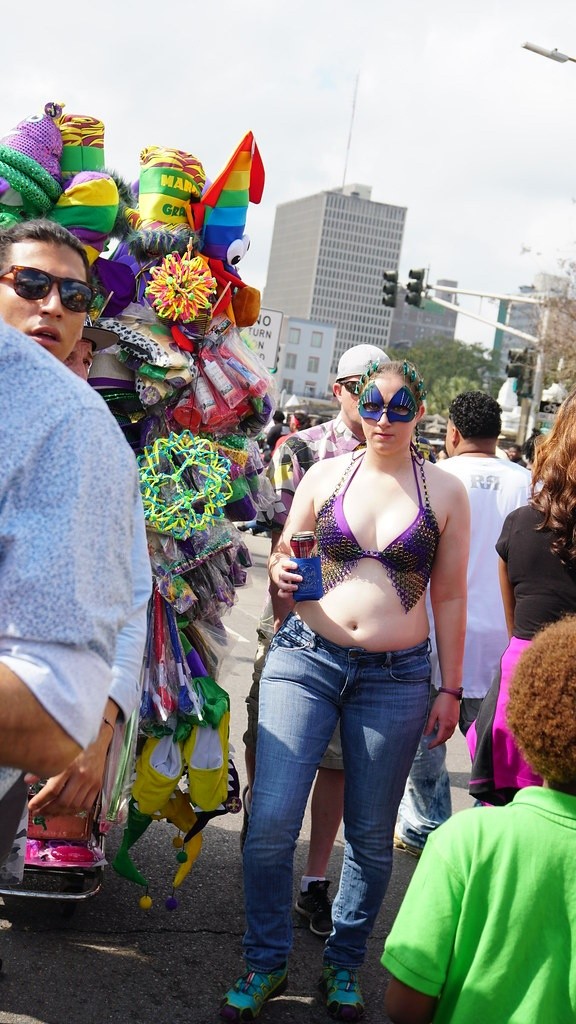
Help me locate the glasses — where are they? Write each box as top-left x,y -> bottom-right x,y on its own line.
0,265 -> 93,313
333,381 -> 361,396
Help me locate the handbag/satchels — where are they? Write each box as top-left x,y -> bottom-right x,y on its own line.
27,782 -> 92,843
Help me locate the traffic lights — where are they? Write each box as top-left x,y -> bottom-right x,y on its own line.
404,268 -> 425,308
506,348 -> 536,397
382,270 -> 398,308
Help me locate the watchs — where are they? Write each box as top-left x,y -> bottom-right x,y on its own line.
439,687 -> 464,700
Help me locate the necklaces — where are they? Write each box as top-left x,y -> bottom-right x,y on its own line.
458,451 -> 496,455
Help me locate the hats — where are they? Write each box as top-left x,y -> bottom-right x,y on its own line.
271,411 -> 287,422
335,344 -> 391,382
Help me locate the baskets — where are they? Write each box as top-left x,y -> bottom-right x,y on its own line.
185,314 -> 213,335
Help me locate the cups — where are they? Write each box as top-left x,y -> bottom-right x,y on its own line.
289,556 -> 322,603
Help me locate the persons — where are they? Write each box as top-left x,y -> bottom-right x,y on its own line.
219,362 -> 471,1024
393,390 -> 544,858
237,411 -> 327,536
380,617 -> 576,1024
241,343 -> 391,934
0,319 -> 134,778
1,220 -> 149,887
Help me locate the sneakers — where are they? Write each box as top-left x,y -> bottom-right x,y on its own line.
392,821 -> 428,857
317,961 -> 365,1017
221,961 -> 288,1024
295,880 -> 333,934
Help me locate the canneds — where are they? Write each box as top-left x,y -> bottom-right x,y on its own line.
290,531 -> 318,559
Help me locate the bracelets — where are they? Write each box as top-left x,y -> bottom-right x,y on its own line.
103,717 -> 115,733
